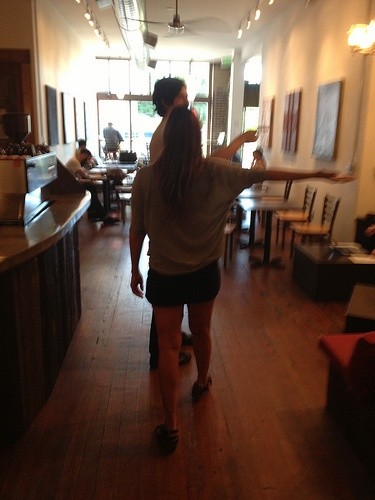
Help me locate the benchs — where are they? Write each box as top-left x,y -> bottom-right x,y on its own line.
317,333 -> 375,469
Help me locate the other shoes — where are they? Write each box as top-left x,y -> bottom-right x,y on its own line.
181,330 -> 194,346
150,348 -> 192,370
154,424 -> 178,446
88,216 -> 103,223
192,374 -> 212,400
104,216 -> 119,224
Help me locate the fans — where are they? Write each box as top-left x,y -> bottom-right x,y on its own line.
123,0 -> 229,38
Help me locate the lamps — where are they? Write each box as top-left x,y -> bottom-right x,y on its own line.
348,18 -> 375,56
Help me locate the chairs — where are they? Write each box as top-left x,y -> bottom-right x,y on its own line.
219,180 -> 340,268
81,152 -> 144,219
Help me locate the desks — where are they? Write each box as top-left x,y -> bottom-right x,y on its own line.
235,187 -> 282,198
234,199 -> 294,265
97,164 -> 135,201
291,241 -> 375,300
343,282 -> 375,336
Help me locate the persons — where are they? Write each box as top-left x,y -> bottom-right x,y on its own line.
149,78 -> 258,369
253,150 -> 264,168
64,139 -> 103,219
129,108 -> 357,449
103,123 -> 123,159
